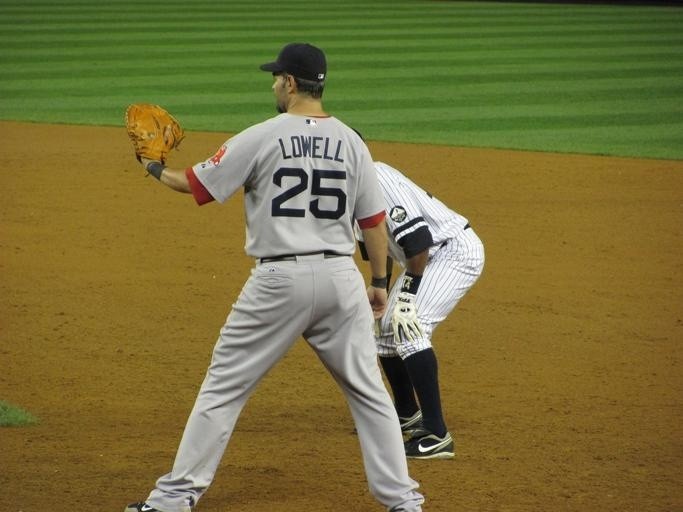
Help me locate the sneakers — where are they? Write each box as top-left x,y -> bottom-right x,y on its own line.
126,501 -> 159,512
405,428 -> 456,461
398,409 -> 424,435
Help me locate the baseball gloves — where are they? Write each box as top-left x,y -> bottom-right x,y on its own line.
124,103 -> 187,164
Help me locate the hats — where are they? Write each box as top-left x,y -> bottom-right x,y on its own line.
259,43 -> 326,82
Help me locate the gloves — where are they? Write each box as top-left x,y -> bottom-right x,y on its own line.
392,272 -> 423,343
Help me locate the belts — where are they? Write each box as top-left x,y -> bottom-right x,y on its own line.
441,222 -> 471,247
261,252 -> 341,262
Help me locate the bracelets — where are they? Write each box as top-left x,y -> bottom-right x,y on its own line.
371,277 -> 388,289
401,272 -> 422,295
147,162 -> 168,180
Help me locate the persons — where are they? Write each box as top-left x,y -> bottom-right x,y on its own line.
124,42 -> 425,512
353,128 -> 486,460
